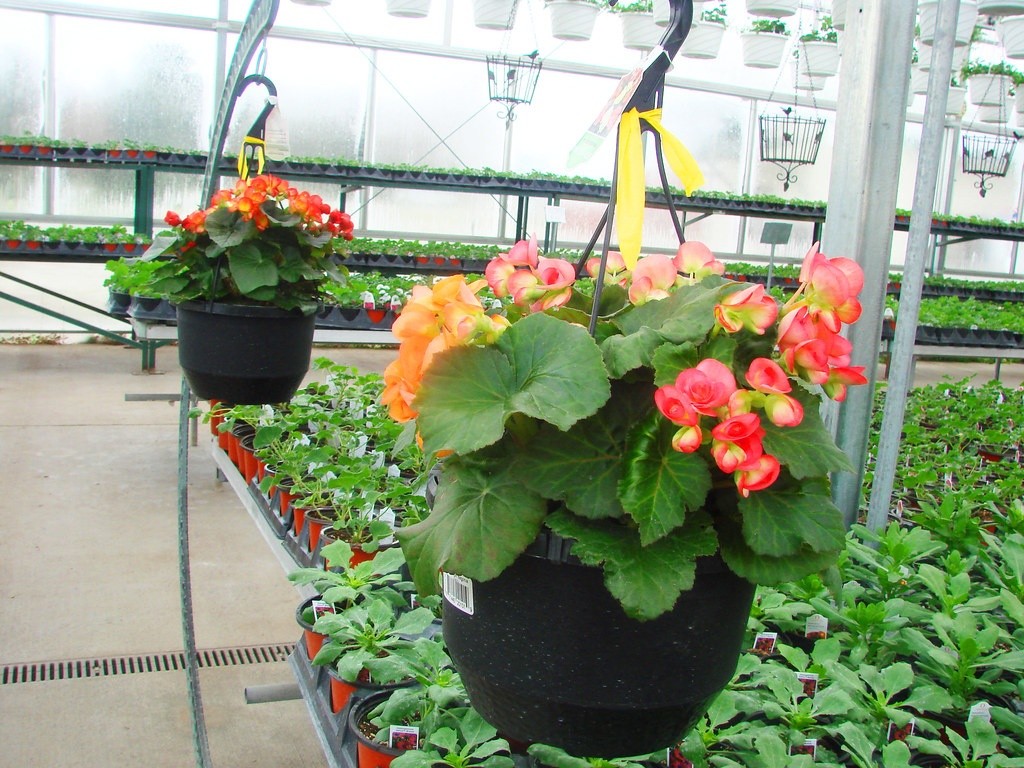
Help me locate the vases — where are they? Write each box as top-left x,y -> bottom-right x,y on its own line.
425,473 -> 756,758
169,298 -> 317,405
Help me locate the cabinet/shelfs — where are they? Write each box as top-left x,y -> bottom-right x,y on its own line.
1,153 -> 1023,371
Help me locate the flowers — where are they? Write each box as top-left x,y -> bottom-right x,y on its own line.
380,233 -> 869,622
164,176 -> 354,316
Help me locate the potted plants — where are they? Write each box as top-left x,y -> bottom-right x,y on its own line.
0,124 -> 1023,343
185,355 -> 1023,768
291,0 -> 1023,124
